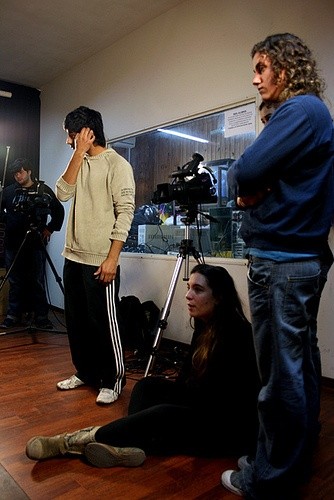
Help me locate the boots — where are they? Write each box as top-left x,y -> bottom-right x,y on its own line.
25,425 -> 102,461
85,441 -> 146,468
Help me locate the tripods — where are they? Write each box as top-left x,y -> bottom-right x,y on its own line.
142,216 -> 203,379
0,221 -> 65,336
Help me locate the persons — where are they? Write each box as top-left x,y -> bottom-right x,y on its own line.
26,265 -> 260,468
222,33 -> 334,500
0,158 -> 65,332
56,107 -> 136,404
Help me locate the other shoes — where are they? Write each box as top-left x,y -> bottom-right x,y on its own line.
0,313 -> 19,328
56,375 -> 85,390
237,454 -> 256,470
221,469 -> 249,497
33,316 -> 53,329
95,387 -> 119,405
